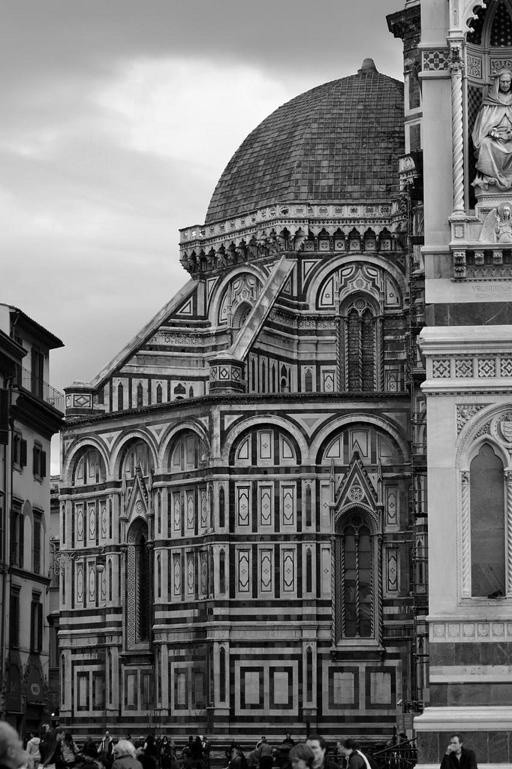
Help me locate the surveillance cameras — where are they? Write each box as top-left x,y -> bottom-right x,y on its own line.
397,699 -> 403,706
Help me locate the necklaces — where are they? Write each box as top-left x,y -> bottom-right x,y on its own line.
456,751 -> 461,755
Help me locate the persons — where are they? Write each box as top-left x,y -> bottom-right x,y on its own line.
385,733 -> 418,768
494,207 -> 512,245
440,734 -> 479,769
0,721 -> 211,769
225,734 -> 377,768
469,67 -> 512,193
